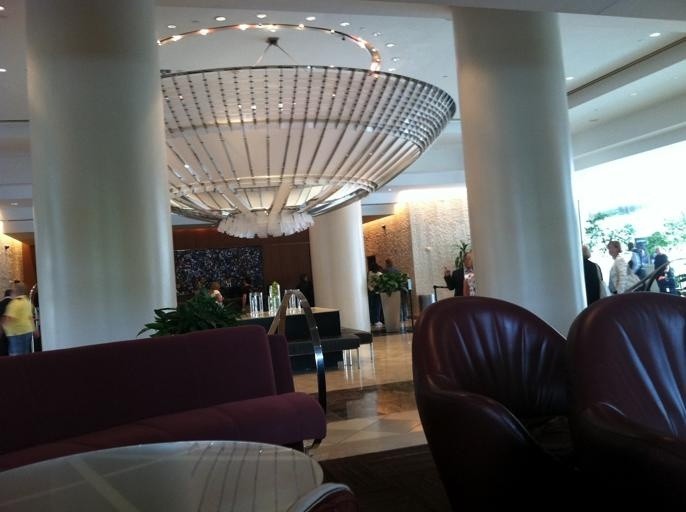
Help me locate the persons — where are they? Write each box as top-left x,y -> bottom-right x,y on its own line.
206,281 -> 224,309
0,279 -> 40,356
583,245 -> 602,305
296,271 -> 310,293
463,272 -> 476,296
241,277 -> 254,314
443,251 -> 473,297
369,260 -> 383,328
607,239 -> 677,295
384,258 -> 398,272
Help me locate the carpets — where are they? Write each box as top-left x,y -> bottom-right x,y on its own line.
319,443 -> 450,511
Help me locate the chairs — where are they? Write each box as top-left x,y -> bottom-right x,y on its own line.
562,294 -> 685,512
410,293 -> 572,512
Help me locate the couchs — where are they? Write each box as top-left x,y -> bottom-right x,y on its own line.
0,290 -> 327,473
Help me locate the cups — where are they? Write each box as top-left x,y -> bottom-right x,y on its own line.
248,291 -> 263,316
267,285 -> 281,316
285,289 -> 302,315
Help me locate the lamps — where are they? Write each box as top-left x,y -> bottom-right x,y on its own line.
155,19 -> 455,239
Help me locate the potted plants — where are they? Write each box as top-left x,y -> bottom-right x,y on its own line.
368,266 -> 410,332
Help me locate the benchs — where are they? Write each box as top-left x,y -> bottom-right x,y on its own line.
287,326 -> 373,357
